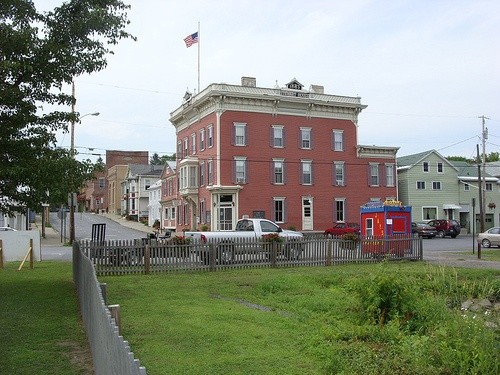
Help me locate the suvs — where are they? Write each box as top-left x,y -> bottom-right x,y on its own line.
427,219 -> 461,239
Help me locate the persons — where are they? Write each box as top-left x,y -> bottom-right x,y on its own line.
153,220 -> 160,235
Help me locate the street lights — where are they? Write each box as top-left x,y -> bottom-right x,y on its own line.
69,113 -> 100,244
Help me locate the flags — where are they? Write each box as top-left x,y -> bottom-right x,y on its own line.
184,32 -> 199,48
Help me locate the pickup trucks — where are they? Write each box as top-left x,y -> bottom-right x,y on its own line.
183,217 -> 305,264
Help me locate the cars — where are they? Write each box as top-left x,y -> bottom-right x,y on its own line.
411,222 -> 438,239
324,222 -> 360,239
478,227 -> 500,248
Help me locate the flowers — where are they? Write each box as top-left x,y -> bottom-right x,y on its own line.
262,233 -> 284,243
171,236 -> 191,246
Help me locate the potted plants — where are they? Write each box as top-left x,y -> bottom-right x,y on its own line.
201,226 -> 208,231
339,234 -> 359,251
